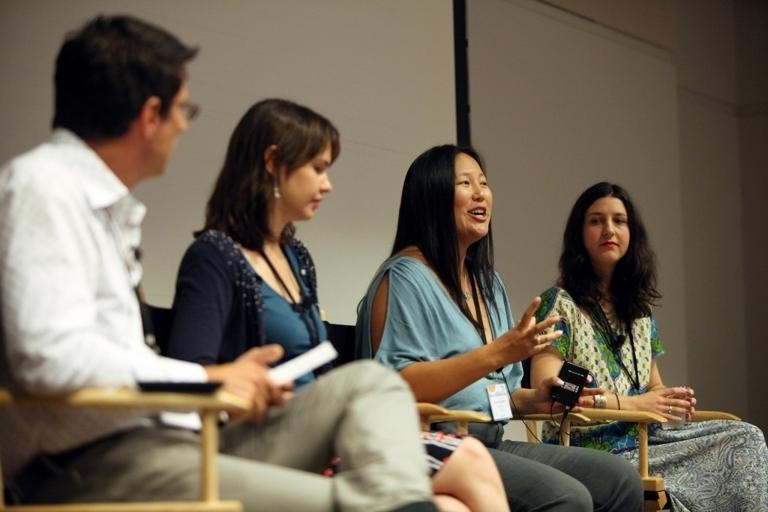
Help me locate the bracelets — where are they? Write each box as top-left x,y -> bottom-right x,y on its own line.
593,391 -> 608,411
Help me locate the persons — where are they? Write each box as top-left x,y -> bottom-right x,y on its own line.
163,98 -> 514,512
0,12 -> 437,512
529,180 -> 764,512
356,143 -> 644,512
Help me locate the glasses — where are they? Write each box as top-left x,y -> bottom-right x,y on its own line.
171,99 -> 202,119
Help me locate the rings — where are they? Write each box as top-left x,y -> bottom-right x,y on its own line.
533,331 -> 547,349
667,403 -> 678,415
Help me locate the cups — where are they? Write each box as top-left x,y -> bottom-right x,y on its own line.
661,409 -> 686,431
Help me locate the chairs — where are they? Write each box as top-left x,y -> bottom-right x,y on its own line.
283,389 -> 454,511
431,401 -> 590,452
524,393 -> 743,512
1,372 -> 248,510
